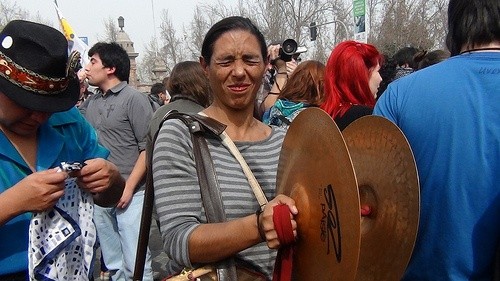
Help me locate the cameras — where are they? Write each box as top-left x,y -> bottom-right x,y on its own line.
279,38 -> 307,61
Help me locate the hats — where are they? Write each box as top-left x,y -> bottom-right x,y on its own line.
0,20 -> 80,112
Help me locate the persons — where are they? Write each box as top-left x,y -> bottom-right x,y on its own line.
261,60 -> 325,126
77,42 -> 153,281
374,47 -> 450,102
0,20 -> 125,281
253,41 -> 307,122
152,16 -> 287,281
143,76 -> 172,114
372,0 -> 500,281
318,40 -> 382,132
151,61 -> 210,139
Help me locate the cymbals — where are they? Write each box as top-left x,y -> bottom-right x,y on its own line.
274,106 -> 361,280
341,114 -> 421,280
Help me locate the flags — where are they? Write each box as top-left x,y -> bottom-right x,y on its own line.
54,5 -> 99,94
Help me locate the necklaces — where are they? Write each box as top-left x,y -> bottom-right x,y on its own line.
459,47 -> 500,54
